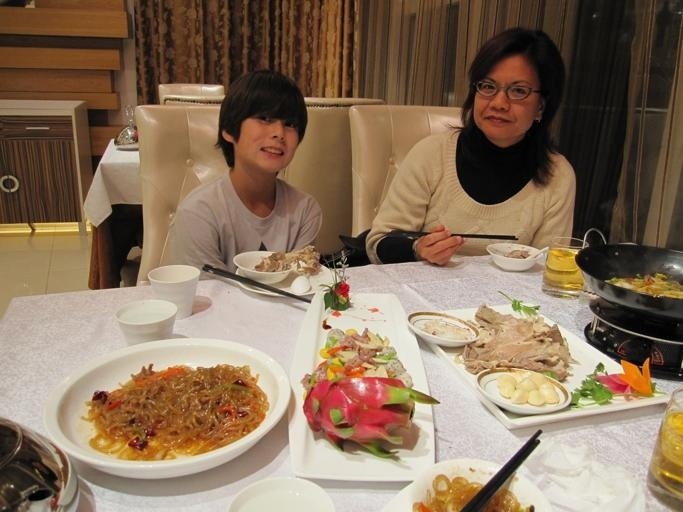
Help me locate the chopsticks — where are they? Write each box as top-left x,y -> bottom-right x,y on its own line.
401,231 -> 520,242
461,429 -> 541,511
202,264 -> 311,306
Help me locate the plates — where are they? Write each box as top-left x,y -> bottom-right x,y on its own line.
286,291 -> 436,484
381,457 -> 552,512
425,302 -> 672,431
407,308 -> 479,348
476,366 -> 572,415
232,261 -> 336,297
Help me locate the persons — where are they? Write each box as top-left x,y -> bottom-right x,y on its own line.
366,27 -> 576,266
158,70 -> 323,280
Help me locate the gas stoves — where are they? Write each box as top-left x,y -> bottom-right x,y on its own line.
583,298 -> 683,380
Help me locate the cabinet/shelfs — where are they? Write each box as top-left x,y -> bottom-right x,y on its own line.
0,99 -> 94,233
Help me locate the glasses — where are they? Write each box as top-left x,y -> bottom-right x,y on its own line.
471,80 -> 542,100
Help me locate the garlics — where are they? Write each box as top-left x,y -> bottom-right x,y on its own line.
496,373 -> 559,406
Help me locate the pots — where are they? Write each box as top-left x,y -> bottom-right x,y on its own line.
575,243 -> 683,319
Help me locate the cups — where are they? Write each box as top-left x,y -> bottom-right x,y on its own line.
646,384 -> 683,511
541,236 -> 590,299
147,265 -> 200,319
115,300 -> 178,345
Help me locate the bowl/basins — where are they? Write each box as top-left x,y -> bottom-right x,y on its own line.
44,337 -> 290,480
234,250 -> 293,285
228,475 -> 335,512
486,243 -> 542,273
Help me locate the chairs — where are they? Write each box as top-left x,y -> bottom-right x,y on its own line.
157,83 -> 226,105
349,104 -> 472,285
129,104 -> 348,283
